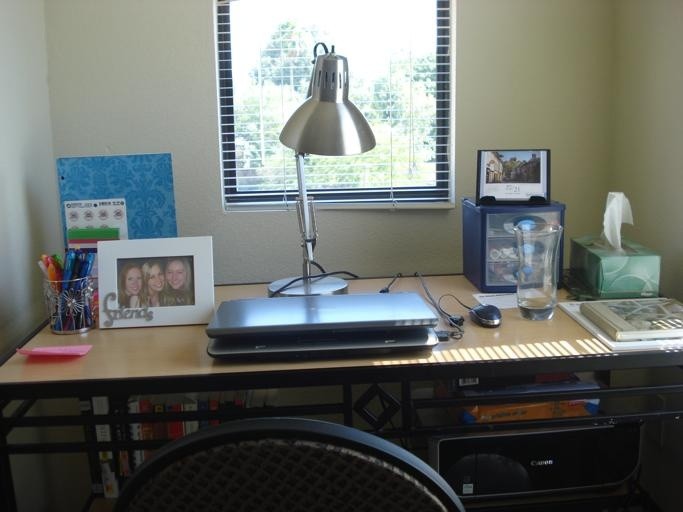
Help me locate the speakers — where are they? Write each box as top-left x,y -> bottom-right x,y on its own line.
426,414 -> 648,505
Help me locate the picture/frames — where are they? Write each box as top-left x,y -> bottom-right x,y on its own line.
96,234 -> 215,329
475,148 -> 552,207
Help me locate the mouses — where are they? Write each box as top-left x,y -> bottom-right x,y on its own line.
468,300 -> 504,329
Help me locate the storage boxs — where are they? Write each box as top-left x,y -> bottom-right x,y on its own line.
460,194 -> 567,294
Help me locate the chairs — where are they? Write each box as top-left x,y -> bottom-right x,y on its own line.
109,415 -> 466,512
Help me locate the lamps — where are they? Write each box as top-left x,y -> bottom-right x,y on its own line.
264,41 -> 378,301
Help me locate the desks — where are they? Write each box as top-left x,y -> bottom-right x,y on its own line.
2,268 -> 683,512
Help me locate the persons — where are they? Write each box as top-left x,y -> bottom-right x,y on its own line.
119,257 -> 194,308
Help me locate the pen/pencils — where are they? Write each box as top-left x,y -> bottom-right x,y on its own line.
40,246 -> 95,332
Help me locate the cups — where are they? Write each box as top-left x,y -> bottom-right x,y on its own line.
514,222 -> 564,320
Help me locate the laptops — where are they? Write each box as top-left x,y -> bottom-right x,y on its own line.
205,288 -> 441,361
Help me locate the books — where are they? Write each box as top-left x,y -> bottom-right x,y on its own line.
557,298 -> 683,352
432,373 -> 614,426
77,389 -> 282,498
